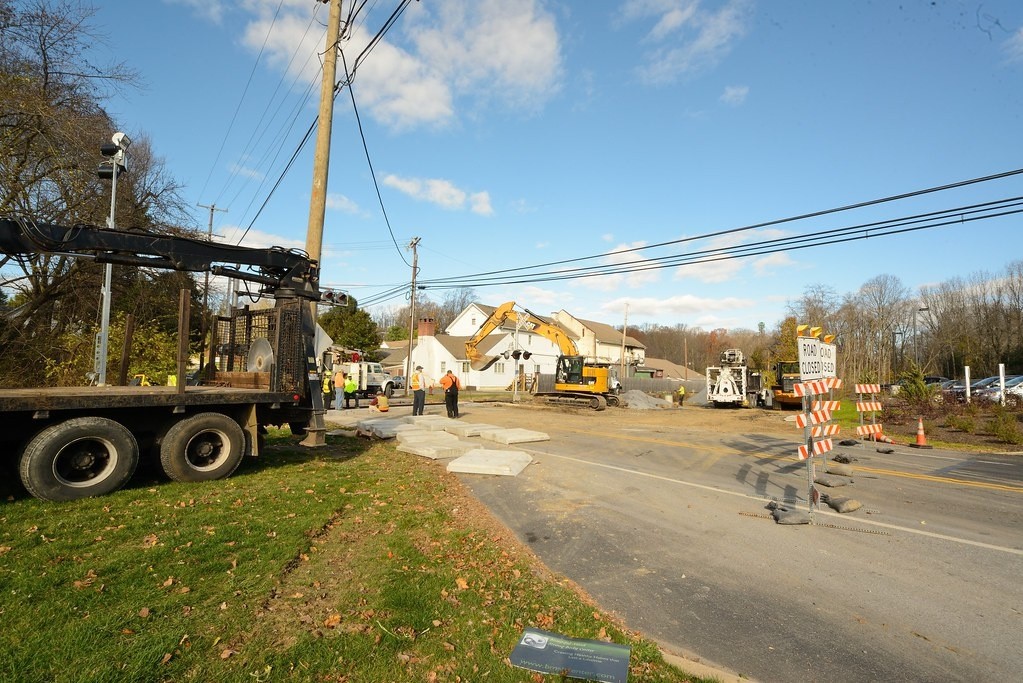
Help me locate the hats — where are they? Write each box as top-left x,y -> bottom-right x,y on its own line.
325,372 -> 332,376
347,373 -> 353,377
416,366 -> 423,370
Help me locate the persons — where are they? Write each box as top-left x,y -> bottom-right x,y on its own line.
439,369 -> 461,418
412,366 -> 426,416
678,383 -> 685,406
345,373 -> 360,409
369,390 -> 390,413
322,371 -> 334,409
334,368 -> 346,409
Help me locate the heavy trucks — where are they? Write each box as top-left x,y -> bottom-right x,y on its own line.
0,216 -> 329,508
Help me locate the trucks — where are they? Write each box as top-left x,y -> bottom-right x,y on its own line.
316,346 -> 396,399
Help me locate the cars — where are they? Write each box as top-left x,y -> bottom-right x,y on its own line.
392,376 -> 406,389
878,375 -> 1023,410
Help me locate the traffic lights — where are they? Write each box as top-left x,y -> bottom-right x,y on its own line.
323,290 -> 346,303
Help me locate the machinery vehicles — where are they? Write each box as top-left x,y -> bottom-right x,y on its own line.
704,348 -> 763,408
464,301 -> 620,413
770,360 -> 803,412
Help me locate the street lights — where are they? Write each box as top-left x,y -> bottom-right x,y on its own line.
92,132 -> 133,389
913,306 -> 932,372
892,331 -> 904,385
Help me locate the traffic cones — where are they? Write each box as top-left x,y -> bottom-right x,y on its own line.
868,429 -> 896,445
910,417 -> 933,450
